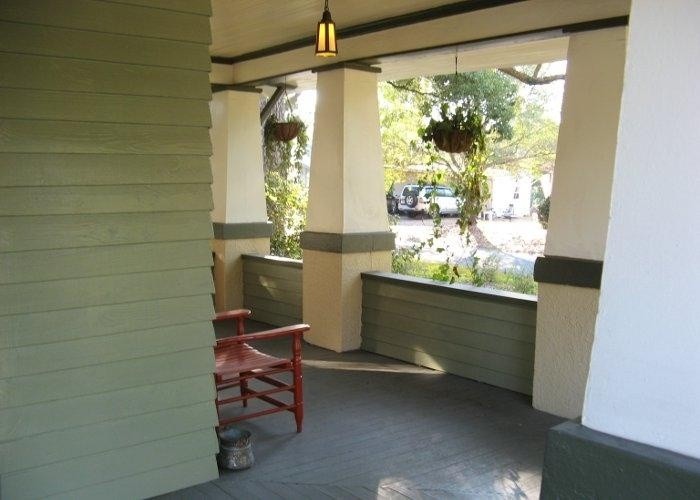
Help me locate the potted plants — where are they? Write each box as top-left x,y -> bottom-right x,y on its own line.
262,114 -> 310,184
417,100 -> 492,247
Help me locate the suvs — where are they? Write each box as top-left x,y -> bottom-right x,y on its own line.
398,184 -> 465,218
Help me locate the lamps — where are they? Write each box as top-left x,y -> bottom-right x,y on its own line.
314,1 -> 339,57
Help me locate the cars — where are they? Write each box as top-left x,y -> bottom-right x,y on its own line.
386,192 -> 398,214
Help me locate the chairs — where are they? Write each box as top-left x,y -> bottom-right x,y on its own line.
212,307 -> 311,438
